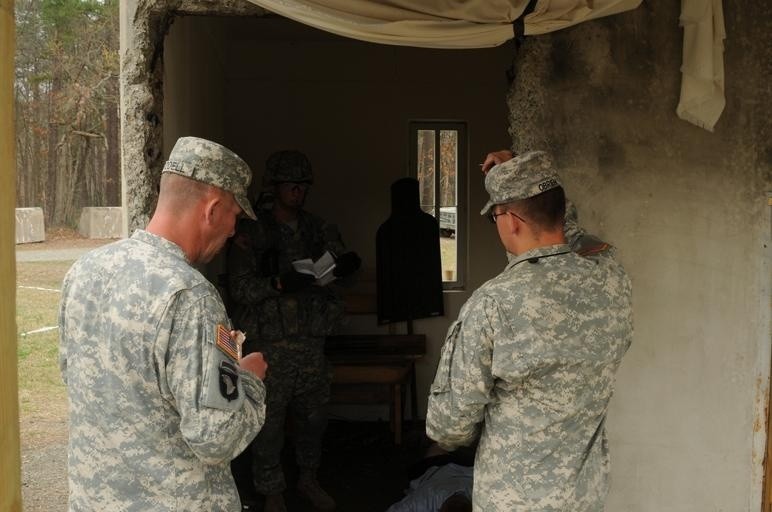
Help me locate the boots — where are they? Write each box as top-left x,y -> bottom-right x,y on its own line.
296,467 -> 336,511
264,492 -> 287,512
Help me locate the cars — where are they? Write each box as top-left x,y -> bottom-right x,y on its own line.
428,205 -> 457,238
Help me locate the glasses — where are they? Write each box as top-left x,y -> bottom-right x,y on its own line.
485,212 -> 527,224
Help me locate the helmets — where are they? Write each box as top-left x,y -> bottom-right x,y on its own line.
262,148 -> 314,187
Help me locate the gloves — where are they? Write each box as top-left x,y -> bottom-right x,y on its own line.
273,269 -> 316,294
332,251 -> 362,277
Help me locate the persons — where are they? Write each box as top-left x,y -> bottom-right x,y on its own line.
56,134 -> 269,512
226,148 -> 351,511
424,147 -> 636,511
385,441 -> 473,511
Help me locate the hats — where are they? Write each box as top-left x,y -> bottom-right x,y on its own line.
478,149 -> 561,215
161,135 -> 259,221
